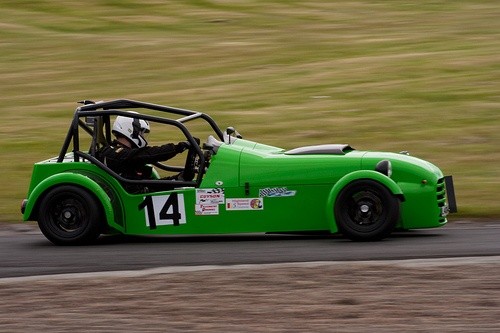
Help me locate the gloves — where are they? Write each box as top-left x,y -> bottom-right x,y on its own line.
177,170 -> 195,182
177,137 -> 200,153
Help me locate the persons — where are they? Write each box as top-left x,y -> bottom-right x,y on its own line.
100,111 -> 200,195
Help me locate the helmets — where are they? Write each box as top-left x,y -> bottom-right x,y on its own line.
112,111 -> 151,149
